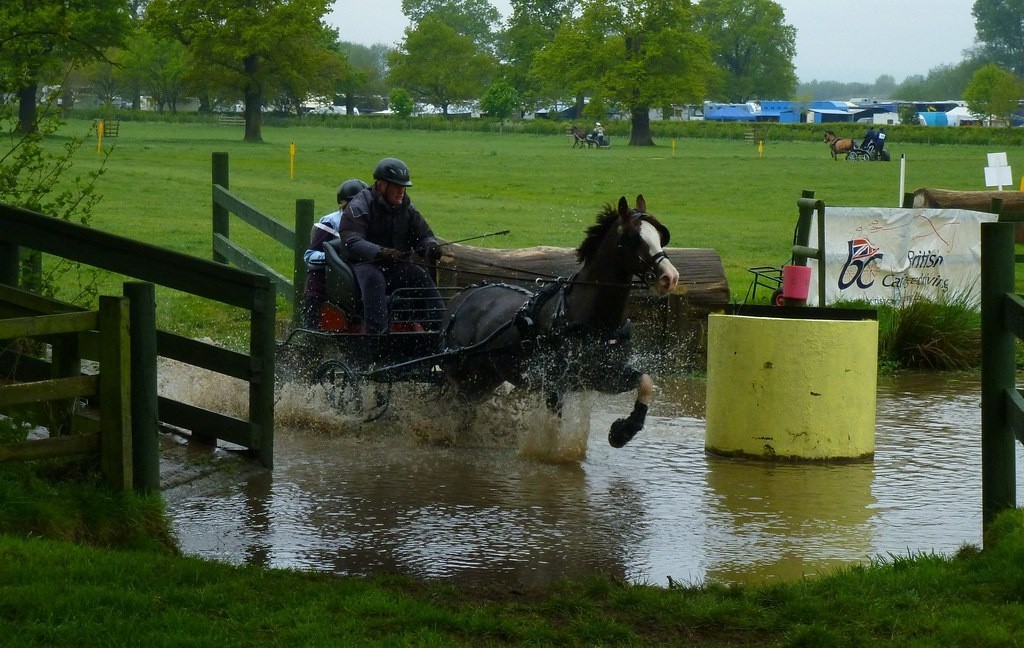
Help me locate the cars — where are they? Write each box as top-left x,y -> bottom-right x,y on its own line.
354,102 -> 378,114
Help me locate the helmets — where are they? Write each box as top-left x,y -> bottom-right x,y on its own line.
336,180 -> 370,204
373,158 -> 413,187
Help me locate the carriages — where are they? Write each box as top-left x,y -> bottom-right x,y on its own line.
824,128 -> 890,163
274,194 -> 680,449
569,126 -> 611,150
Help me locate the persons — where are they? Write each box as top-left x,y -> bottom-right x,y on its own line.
593,122 -> 606,145
861,125 -> 886,160
339,158 -> 448,331
303,179 -> 369,328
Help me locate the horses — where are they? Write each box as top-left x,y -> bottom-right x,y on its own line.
570,126 -> 593,149
434,194 -> 680,448
823,129 -> 857,161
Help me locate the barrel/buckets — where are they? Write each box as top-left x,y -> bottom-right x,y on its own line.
782,265 -> 812,300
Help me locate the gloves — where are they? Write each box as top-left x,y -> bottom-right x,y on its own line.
426,242 -> 441,261
377,248 -> 405,261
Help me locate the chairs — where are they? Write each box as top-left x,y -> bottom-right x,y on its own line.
322,238 -> 420,321
870,142 -> 884,151
